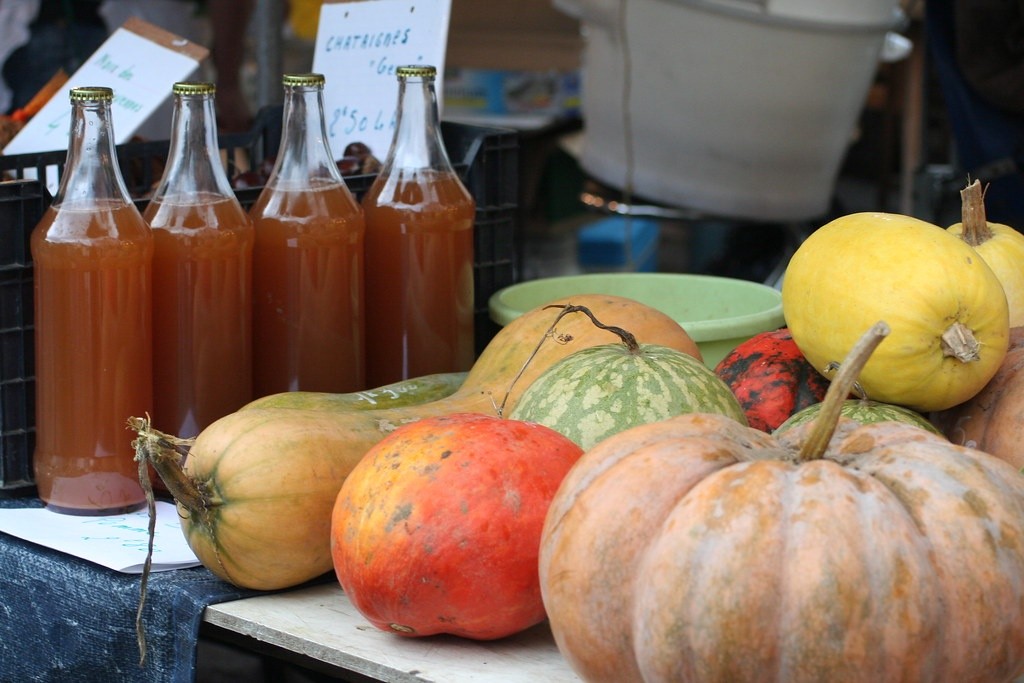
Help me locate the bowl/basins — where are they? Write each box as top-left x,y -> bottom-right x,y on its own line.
487,272 -> 782,374
552,1 -> 901,221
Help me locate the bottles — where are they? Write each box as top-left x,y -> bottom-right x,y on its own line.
143,84 -> 253,503
32,87 -> 154,515
249,73 -> 366,398
361,64 -> 475,390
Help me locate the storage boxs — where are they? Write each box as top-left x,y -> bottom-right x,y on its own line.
1,118 -> 524,509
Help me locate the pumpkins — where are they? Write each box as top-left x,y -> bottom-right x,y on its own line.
126,181 -> 1024,683
